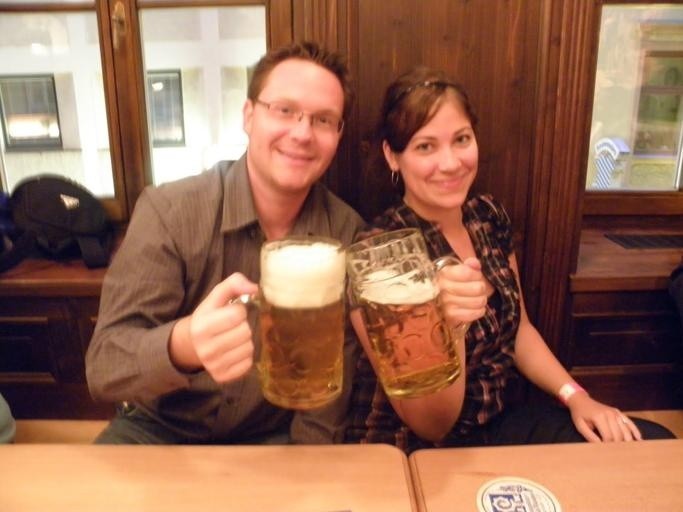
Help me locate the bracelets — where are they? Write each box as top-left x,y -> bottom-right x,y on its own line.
558,382 -> 586,405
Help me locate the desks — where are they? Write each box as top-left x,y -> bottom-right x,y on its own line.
0,438 -> 420,511
409,437 -> 682,511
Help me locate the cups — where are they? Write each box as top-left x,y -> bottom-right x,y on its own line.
226,233 -> 347,412
346,227 -> 466,400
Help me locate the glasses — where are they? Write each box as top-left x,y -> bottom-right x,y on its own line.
250,96 -> 345,135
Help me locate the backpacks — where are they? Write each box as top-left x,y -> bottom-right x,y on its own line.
0,174 -> 111,273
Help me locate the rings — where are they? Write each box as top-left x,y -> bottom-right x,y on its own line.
622,414 -> 630,422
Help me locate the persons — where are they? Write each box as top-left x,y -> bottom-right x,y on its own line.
85,42 -> 369,445
344,65 -> 676,458
